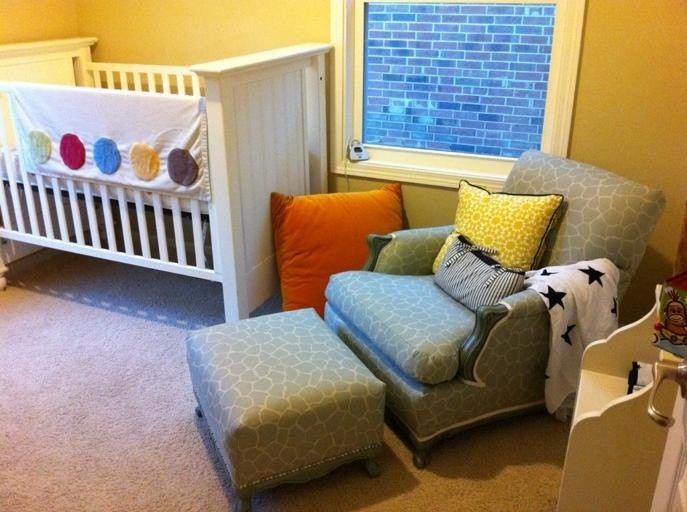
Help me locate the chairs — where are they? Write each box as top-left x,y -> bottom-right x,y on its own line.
323,149 -> 667,468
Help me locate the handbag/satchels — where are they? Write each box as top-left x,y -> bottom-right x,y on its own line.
433,235 -> 525,311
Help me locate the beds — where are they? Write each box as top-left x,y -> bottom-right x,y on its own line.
0,37 -> 334,324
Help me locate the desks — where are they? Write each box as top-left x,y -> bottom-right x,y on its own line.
556,284 -> 684,512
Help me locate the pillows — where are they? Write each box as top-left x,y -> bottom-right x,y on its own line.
431,181 -> 565,276
270,180 -> 407,320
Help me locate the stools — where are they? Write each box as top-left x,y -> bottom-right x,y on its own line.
185,306 -> 387,512
